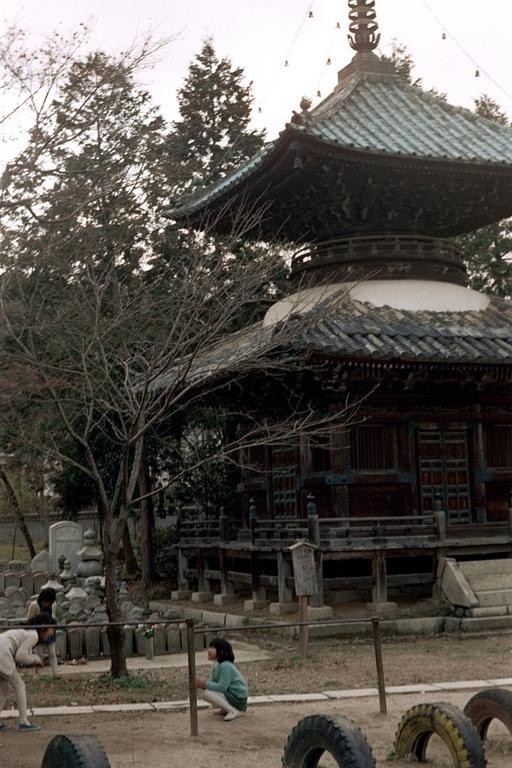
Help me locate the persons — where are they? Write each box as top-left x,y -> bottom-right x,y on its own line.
0,614 -> 57,732
196,638 -> 247,721
29,587 -> 62,681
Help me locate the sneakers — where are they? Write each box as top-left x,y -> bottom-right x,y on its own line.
19,722 -> 41,732
0,723 -> 8,730
213,709 -> 245,721
